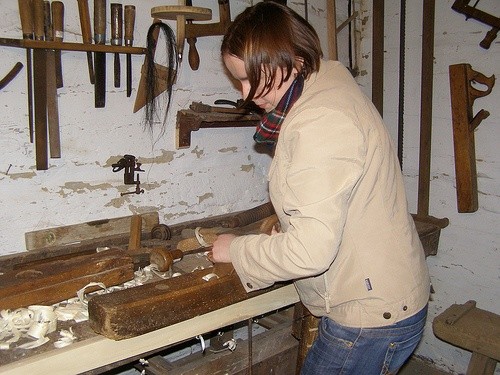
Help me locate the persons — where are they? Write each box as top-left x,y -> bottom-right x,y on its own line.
210,1 -> 432,375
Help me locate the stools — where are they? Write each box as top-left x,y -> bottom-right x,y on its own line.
433,304 -> 500,375
